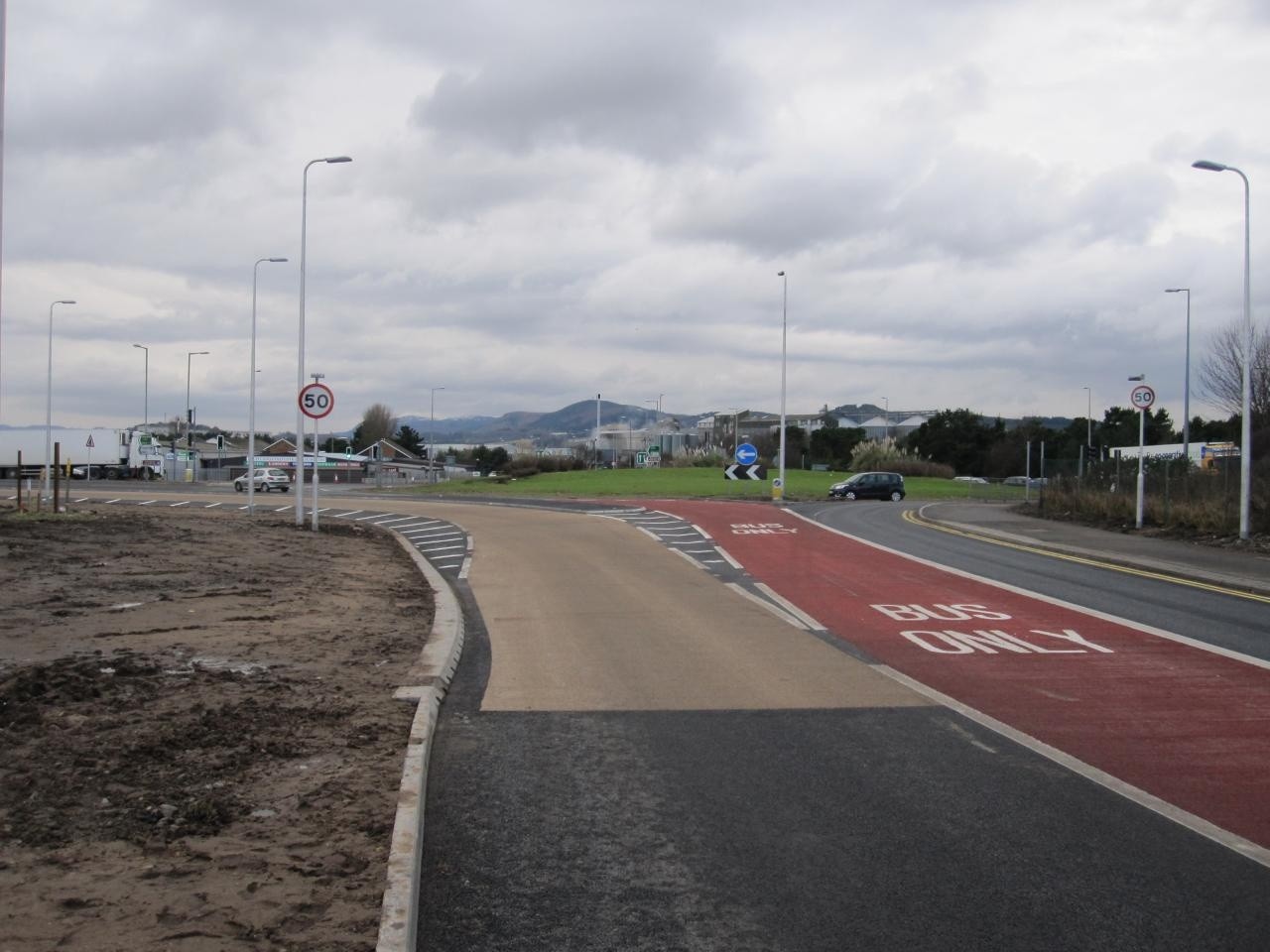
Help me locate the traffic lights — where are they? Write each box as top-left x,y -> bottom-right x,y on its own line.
1087,446 -> 1097,461
217,435 -> 224,451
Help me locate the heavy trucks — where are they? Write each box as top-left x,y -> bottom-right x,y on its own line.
0,428 -> 165,479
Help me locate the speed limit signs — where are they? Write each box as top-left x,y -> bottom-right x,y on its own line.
1131,386 -> 1156,409
299,384 -> 333,418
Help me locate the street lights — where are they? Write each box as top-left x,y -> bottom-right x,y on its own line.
1128,373 -> 1147,528
248,259 -> 289,507
310,372 -> 325,530
292,154 -> 353,523
132,344 -> 148,430
429,387 -> 446,486
880,397 -> 887,448
185,351 -> 213,468
1192,159 -> 1253,543
775,271 -> 788,489
43,299 -> 78,497
1083,386 -> 1092,447
1164,287 -> 1191,457
644,399 -> 659,432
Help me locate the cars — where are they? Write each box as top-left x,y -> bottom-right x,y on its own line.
1003,476 -> 1049,487
953,476 -> 989,485
234,468 -> 292,493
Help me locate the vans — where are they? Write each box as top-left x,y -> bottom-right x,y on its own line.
827,472 -> 906,501
